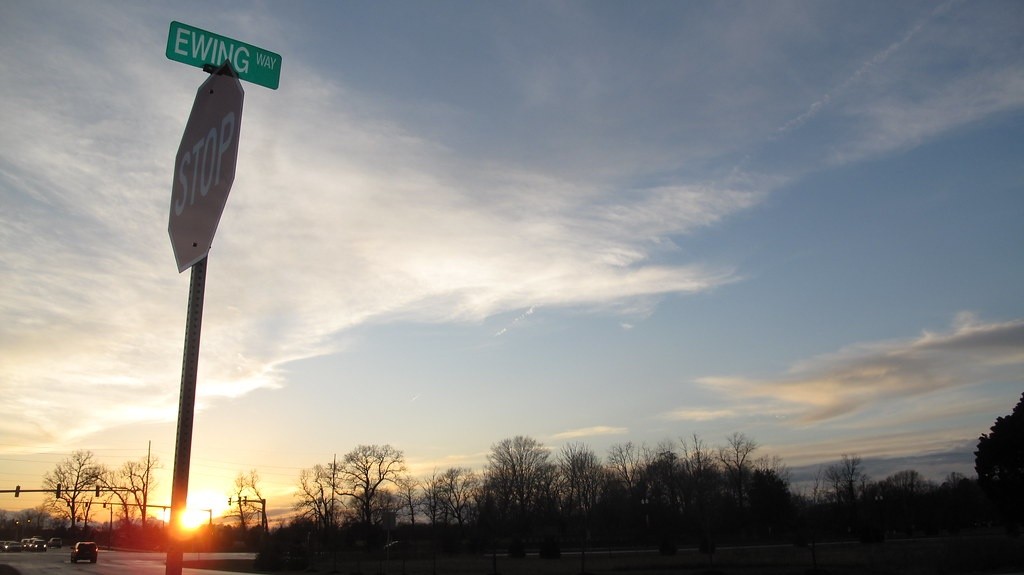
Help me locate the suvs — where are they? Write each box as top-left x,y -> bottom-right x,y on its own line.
48,537 -> 62,549
70,542 -> 99,563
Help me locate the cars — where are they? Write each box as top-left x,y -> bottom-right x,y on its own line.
0,536 -> 47,552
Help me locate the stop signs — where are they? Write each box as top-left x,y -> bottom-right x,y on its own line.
168,59 -> 244,273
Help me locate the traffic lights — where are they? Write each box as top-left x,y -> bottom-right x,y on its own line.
238,497 -> 241,505
228,498 -> 231,505
103,500 -> 107,508
243,496 -> 246,506
67,498 -> 70,506
